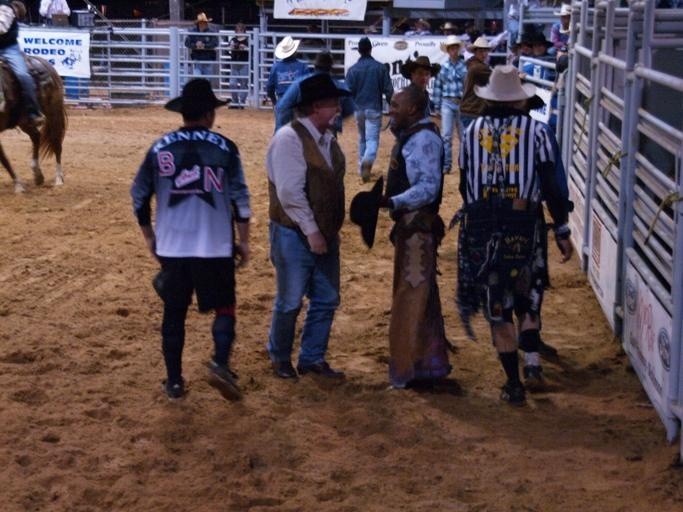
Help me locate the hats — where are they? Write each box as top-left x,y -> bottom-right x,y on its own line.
474,65 -> 535,102
554,4 -> 572,16
526,33 -> 553,48
195,13 -> 212,24
274,36 -> 300,59
440,34 -> 492,53
350,176 -> 384,249
288,74 -> 351,107
165,79 -> 231,114
401,56 -> 440,79
415,19 -> 456,30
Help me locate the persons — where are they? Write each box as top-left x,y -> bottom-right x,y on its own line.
128,79 -> 253,400
447,63 -> 573,404
349,88 -> 456,393
390,56 -> 440,138
263,75 -> 352,382
0,1 -> 44,123
489,78 -> 571,356
403,1 -> 571,174
266,34 -> 308,136
345,38 -> 394,184
38,0 -> 70,26
274,52 -> 353,139
185,13 -> 218,82
226,23 -> 248,109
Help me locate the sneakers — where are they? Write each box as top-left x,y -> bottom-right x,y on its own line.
206,359 -> 242,401
274,362 -> 342,379
500,365 -> 542,406
164,380 -> 184,401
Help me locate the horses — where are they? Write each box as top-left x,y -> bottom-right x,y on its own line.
0,56 -> 67,194
